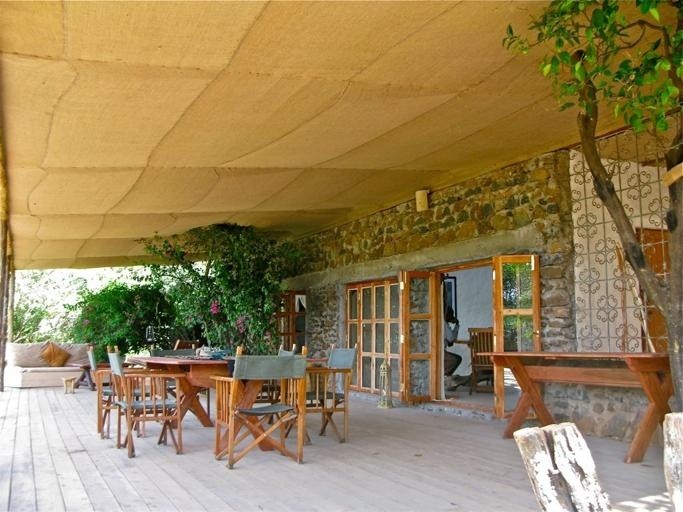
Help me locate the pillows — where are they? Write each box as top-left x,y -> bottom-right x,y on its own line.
40,344 -> 73,367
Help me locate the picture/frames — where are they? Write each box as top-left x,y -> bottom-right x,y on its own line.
442,276 -> 457,322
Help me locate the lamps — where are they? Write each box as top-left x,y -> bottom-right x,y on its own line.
415,189 -> 430,213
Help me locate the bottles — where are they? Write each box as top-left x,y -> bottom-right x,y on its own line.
198,344 -> 224,356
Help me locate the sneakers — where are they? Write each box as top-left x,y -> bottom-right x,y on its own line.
444,375 -> 457,388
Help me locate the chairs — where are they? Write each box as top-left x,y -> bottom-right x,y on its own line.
87,339 -> 358,469
468,326 -> 494,395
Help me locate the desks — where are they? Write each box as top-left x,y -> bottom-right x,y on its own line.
475,352 -> 674,463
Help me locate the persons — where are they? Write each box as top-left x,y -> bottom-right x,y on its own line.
445,305 -> 462,376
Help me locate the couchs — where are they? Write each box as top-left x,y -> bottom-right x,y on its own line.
2,342 -> 88,389
